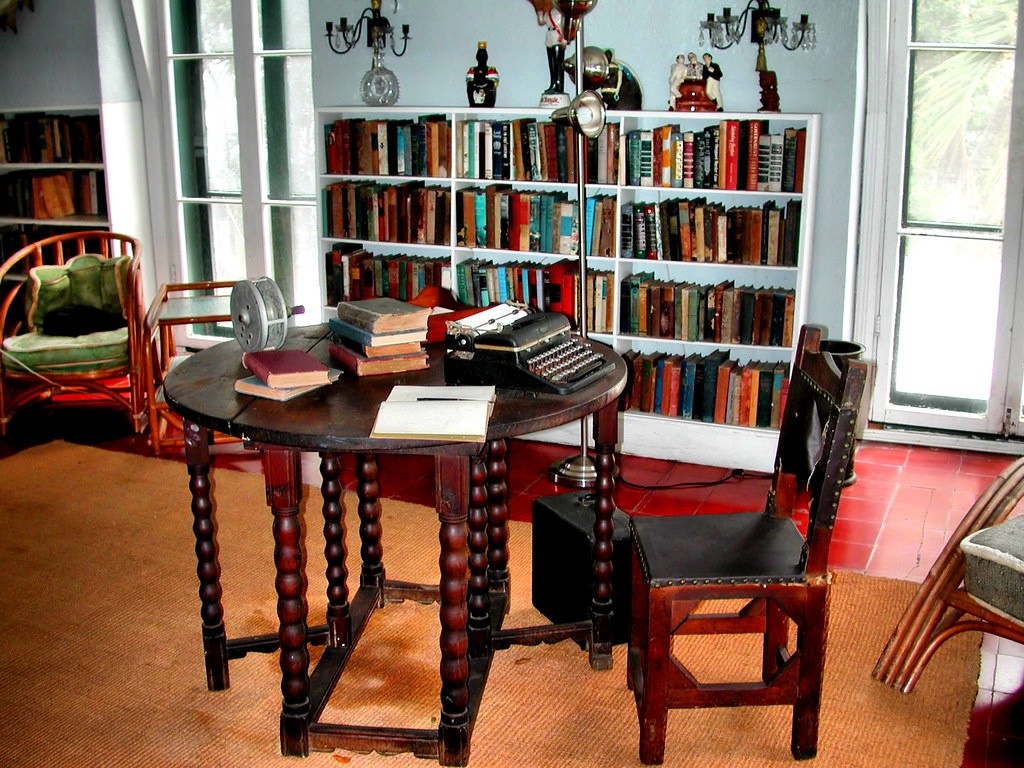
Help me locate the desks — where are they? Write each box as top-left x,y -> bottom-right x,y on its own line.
165,323 -> 631,767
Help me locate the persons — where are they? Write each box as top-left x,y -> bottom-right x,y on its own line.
667,52 -> 726,113
600,49 -> 623,111
535,0 -> 570,94
466,40 -> 500,108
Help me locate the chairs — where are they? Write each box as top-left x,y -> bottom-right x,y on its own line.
626,324 -> 868,765
871,458 -> 1024,693
0,230 -> 163,435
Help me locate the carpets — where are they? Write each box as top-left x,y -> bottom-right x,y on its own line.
0,440 -> 986,768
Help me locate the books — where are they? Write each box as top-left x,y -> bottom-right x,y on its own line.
621,119 -> 808,268
323,242 -> 452,311
321,113 -> 452,247
243,348 -> 333,388
368,386 -> 498,443
456,258 -> 616,337
0,111 -> 113,276
327,297 -> 433,376
615,269 -> 797,429
234,367 -> 344,402
453,117 -> 621,262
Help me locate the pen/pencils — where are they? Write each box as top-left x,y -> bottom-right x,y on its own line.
417,397 -> 497,403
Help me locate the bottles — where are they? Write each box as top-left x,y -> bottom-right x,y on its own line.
360,27 -> 399,107
467,42 -> 497,108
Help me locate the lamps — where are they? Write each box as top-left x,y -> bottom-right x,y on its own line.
325,0 -> 413,69
546,0 -> 620,488
698,0 -> 817,71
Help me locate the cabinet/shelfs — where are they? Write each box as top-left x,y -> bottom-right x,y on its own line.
143,281 -> 243,455
0,100 -> 156,315
313,106 -> 823,472
